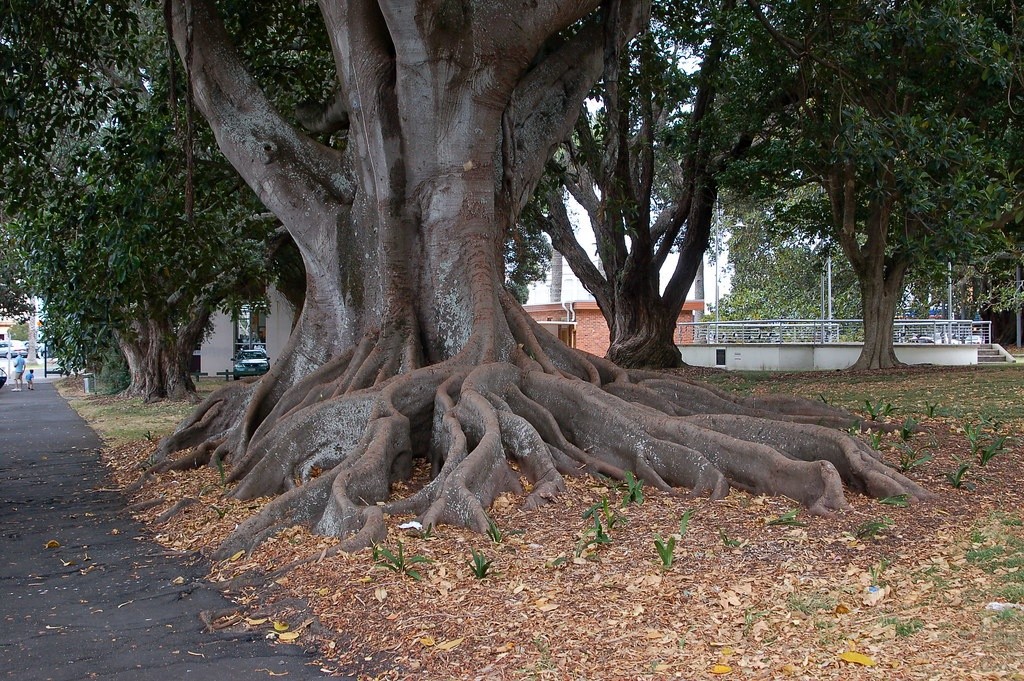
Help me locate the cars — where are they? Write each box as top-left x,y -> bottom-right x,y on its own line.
903,337 -> 962,345
230,350 -> 270,380
0,340 -> 48,358
965,336 -> 981,344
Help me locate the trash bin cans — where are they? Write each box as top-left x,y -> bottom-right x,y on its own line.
82,373 -> 96,394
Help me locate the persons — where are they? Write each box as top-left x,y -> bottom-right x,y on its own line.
26,370 -> 34,390
11,352 -> 26,391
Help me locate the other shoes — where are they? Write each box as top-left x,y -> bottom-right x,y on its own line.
12,388 -> 22,392
28,387 -> 30,389
31,388 -> 34,390
